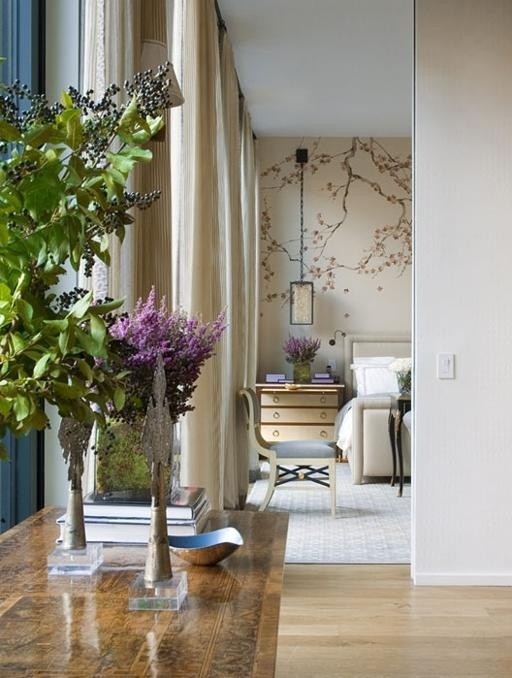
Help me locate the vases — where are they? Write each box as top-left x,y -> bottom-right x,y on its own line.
294,361 -> 311,383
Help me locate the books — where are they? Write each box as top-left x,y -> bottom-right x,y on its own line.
55,487 -> 211,545
313,370 -> 337,384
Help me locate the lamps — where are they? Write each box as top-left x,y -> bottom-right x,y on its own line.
289,148 -> 314,325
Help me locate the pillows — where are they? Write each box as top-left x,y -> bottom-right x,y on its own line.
350,356 -> 411,396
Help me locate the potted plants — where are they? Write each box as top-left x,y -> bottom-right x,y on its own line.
395,357 -> 411,394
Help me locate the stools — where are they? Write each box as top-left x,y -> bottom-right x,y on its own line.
388,395 -> 411,497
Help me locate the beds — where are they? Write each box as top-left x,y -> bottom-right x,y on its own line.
344,332 -> 412,485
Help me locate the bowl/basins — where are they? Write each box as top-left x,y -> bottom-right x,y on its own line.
167,527 -> 244,565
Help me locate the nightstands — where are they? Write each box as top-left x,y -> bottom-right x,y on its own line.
255,383 -> 345,462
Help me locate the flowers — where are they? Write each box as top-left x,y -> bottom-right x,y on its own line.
93,286 -> 227,426
281,335 -> 321,363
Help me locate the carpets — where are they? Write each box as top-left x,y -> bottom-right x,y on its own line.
244,457 -> 409,564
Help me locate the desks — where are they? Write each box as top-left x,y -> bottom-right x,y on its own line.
0,509 -> 290,678
